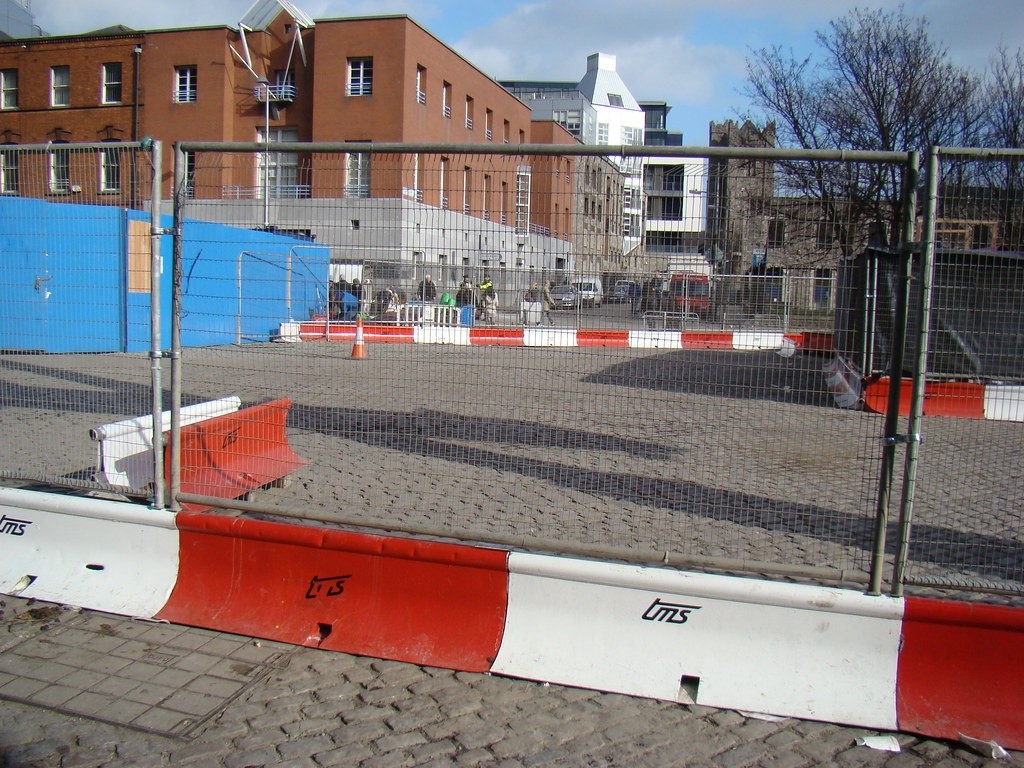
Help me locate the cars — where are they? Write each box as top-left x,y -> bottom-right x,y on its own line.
603,286 -> 641,304
550,286 -> 582,310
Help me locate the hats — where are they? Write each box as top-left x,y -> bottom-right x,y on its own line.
484,275 -> 490,278
339,274 -> 346,282
463,274 -> 469,278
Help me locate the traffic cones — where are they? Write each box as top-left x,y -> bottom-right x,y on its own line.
346,317 -> 371,360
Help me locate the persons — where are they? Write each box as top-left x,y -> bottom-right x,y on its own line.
457,274 -> 472,307
475,275 -> 493,320
628,270 -> 663,315
329,274 -> 407,324
527,280 -> 540,301
417,274 -> 436,301
537,280 -> 555,326
482,286 -> 499,325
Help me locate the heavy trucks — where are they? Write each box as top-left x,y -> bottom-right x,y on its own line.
641,273 -> 710,318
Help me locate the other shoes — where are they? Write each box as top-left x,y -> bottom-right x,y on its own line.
552,323 -> 555,326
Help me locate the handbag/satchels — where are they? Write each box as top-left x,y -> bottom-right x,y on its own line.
525,292 -> 533,302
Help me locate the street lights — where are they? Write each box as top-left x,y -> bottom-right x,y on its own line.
707,204 -> 728,322
690,189 -> 719,322
257,76 -> 269,230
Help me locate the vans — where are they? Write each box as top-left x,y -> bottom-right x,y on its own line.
570,278 -> 603,308
